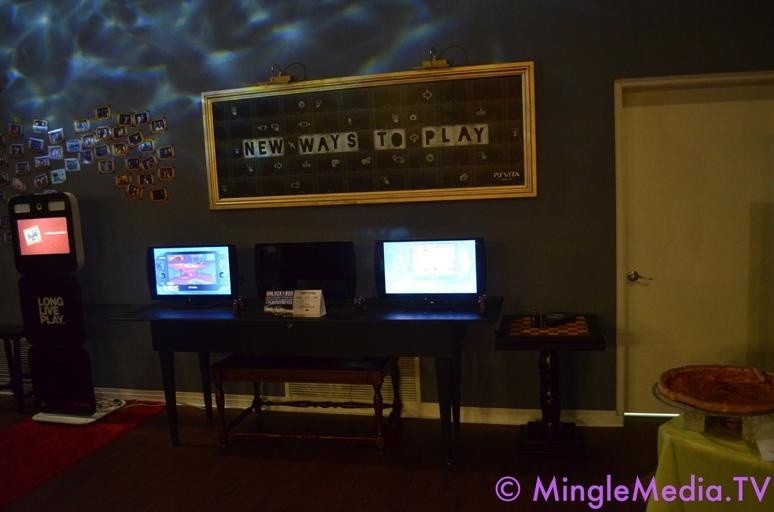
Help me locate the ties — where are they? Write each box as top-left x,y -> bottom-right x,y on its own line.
147,245 -> 237,310
380,238 -> 482,310
254,242 -> 355,302
13,216 -> 75,261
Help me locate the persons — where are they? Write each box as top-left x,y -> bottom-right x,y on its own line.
0,106 -> 174,201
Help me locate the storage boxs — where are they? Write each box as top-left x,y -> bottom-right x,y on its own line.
213,352 -> 403,453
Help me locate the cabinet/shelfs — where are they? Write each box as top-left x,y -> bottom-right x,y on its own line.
478,294 -> 488,313
233,299 -> 240,313
529,309 -> 544,327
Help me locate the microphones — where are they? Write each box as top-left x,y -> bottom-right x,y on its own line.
0,325 -> 32,413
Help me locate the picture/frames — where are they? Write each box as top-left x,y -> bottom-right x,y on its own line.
647,410 -> 774,512
499,314 -> 608,451
108,294 -> 507,455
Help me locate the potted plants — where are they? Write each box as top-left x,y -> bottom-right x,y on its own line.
8,104 -> 176,202
201,58 -> 537,210
